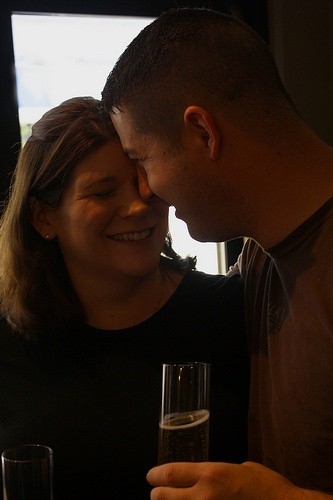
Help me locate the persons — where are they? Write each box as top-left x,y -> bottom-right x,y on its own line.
102,7 -> 333,499
0,97 -> 254,500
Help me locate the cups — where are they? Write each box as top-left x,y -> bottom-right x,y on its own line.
1,444 -> 55,500
156,361 -> 210,468
